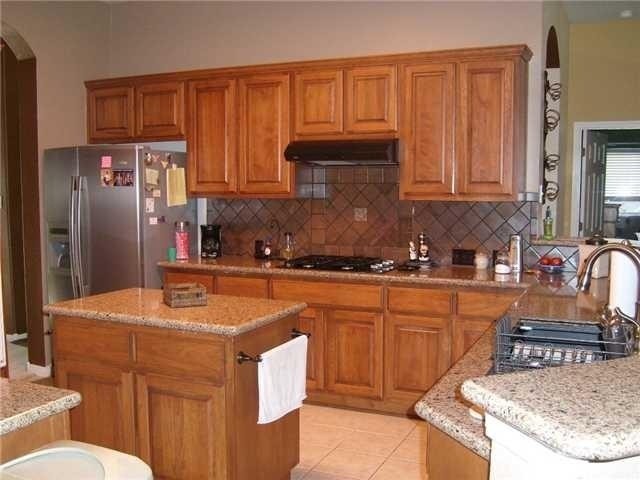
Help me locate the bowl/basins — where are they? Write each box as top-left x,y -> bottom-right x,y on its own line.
537,264 -> 564,274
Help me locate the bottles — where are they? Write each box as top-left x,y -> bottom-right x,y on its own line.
542,204 -> 554,240
510,235 -> 523,272
174,222 -> 189,259
263,239 -> 275,257
418,231 -> 430,263
493,252 -> 512,275
279,232 -> 295,262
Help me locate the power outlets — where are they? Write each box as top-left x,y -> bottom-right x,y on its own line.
353,207 -> 368,222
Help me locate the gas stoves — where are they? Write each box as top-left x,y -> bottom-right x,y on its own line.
276,254 -> 399,274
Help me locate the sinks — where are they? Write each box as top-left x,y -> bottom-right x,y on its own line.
486,316 -> 608,379
0,437 -> 155,479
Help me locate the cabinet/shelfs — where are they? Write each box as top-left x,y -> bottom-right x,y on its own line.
86,69 -> 186,143
162,268 -> 215,303
271,279 -> 384,413
410,406 -> 490,480
52,312 -> 311,480
1,377 -> 85,466
292,57 -> 398,140
384,284 -> 523,413
215,272 -> 270,298
185,61 -> 292,198
396,45 -> 534,203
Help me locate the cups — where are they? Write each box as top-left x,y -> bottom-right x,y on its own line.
167,248 -> 176,263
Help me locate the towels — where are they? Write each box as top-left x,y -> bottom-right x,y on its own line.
256,334 -> 309,425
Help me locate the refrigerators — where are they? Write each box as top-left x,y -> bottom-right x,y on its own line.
43,145 -> 199,306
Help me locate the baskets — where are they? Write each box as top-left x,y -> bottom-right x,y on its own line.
495,313 -> 635,372
164,282 -> 207,307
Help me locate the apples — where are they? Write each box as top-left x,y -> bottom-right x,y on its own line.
540,258 -> 550,265
551,257 -> 562,266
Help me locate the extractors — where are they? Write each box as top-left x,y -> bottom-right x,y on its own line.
285,140 -> 401,167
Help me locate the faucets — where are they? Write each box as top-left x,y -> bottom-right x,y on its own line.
576,239 -> 640,356
